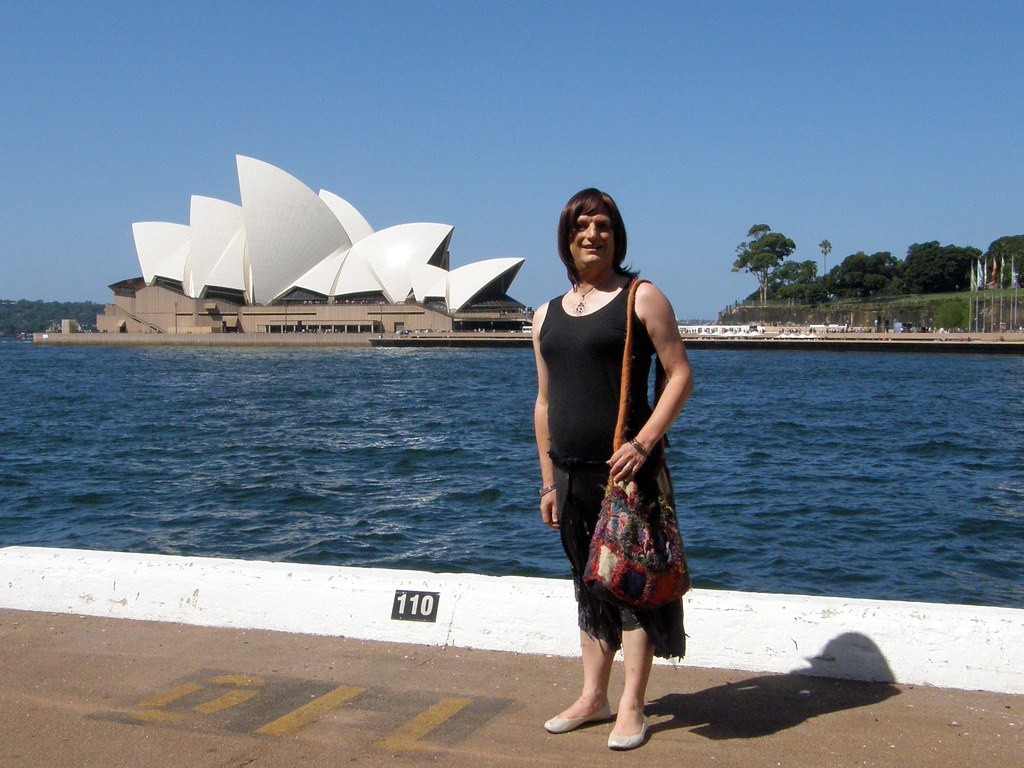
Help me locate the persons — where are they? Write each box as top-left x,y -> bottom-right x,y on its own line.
531,188 -> 694,750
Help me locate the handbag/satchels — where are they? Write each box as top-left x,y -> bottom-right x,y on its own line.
584,470 -> 691,610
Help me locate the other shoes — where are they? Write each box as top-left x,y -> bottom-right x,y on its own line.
544,700 -> 611,733
608,713 -> 649,750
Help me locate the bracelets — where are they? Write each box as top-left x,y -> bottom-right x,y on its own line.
628,437 -> 650,458
540,481 -> 556,496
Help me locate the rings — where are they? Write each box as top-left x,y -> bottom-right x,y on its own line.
626,463 -> 632,470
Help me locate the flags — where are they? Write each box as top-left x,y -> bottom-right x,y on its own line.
970,258 -> 976,292
1011,255 -> 1020,288
983,259 -> 989,287
976,259 -> 983,289
1000,256 -> 1008,284
990,258 -> 997,286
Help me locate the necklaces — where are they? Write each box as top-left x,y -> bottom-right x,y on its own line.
571,273 -> 615,315
